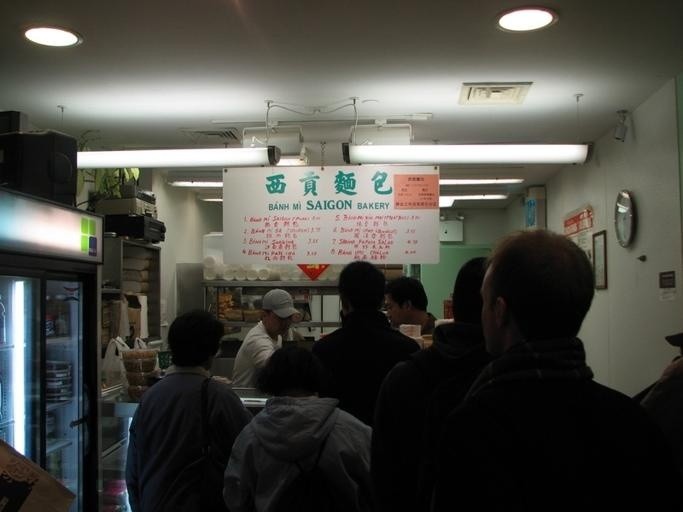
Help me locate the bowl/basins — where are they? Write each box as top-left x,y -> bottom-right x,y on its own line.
120,348 -> 157,401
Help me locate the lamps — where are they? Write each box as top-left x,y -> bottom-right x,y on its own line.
439,177 -> 524,202
165,153 -> 309,203
70,100 -> 280,169
342,93 -> 595,164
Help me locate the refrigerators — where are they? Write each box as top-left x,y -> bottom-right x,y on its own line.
1,186 -> 105,511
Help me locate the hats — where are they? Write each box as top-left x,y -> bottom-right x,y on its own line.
262,289 -> 301,319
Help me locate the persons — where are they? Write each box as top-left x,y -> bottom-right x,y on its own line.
126,230 -> 681,511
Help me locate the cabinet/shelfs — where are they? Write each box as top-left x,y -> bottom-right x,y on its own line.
443,299 -> 453,318
201,280 -> 342,377
104,236 -> 161,342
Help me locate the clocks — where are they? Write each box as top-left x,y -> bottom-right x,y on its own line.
615,189 -> 635,247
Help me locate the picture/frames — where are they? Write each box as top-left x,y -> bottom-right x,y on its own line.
592,230 -> 607,289
